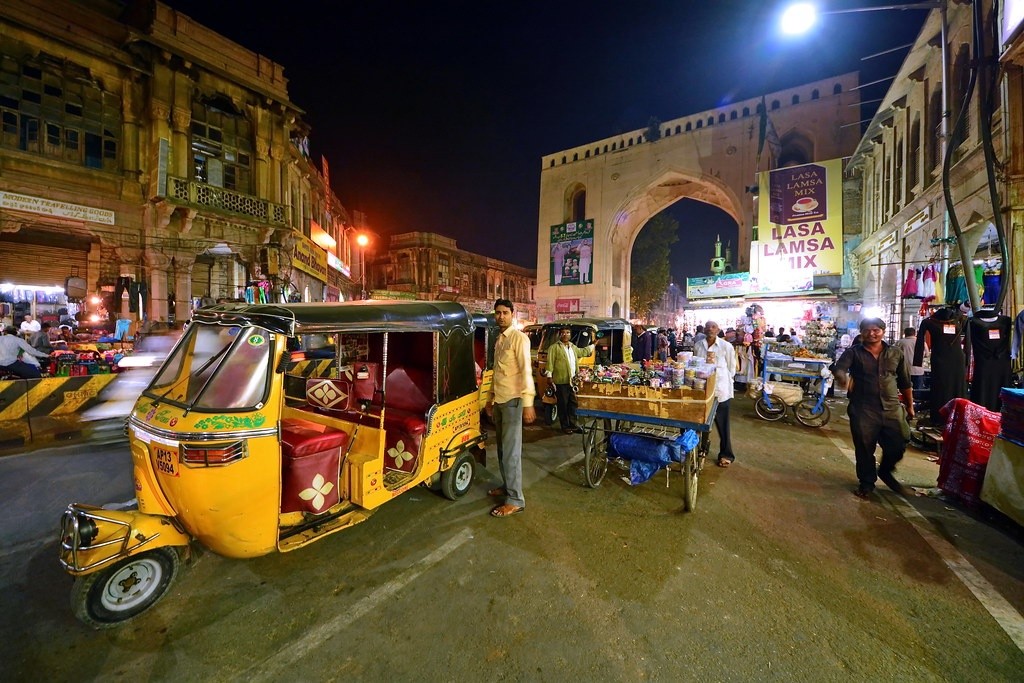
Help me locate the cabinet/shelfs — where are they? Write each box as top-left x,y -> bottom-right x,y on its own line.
763,344 -> 832,388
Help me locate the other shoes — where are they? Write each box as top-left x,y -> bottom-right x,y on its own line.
877,471 -> 901,491
575,426 -> 588,434
855,483 -> 876,496
562,428 -> 574,434
719,458 -> 731,467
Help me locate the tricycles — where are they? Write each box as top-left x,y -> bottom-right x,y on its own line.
581,397 -> 719,512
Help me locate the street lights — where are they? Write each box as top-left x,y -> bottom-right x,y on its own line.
357,233 -> 370,300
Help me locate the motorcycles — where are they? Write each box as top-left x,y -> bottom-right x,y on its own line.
536,317 -> 634,425
0,353 -> 55,381
470,312 -> 499,424
522,324 -> 544,384
57,298 -> 489,631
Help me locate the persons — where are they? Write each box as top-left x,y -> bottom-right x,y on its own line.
654,327 -> 677,361
682,328 -> 693,342
58,326 -> 81,342
835,318 -> 914,495
776,327 -> 802,344
892,328 -> 929,389
632,325 -> 651,362
29,323 -> 54,353
0,326 -> 52,379
542,326 -> 599,434
552,239 -> 593,285
486,298 -> 537,517
693,321 -> 736,467
20,313 -> 41,332
765,330 -> 773,337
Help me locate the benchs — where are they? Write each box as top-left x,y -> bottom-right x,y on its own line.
351,363 -> 433,472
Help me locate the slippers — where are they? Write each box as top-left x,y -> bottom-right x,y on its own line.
487,487 -> 505,495
491,504 -> 525,517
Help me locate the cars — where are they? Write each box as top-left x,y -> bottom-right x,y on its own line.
117,330 -> 184,374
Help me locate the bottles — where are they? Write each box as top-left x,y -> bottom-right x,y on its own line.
664,350 -> 716,390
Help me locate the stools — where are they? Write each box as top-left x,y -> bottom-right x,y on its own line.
280,416 -> 347,516
